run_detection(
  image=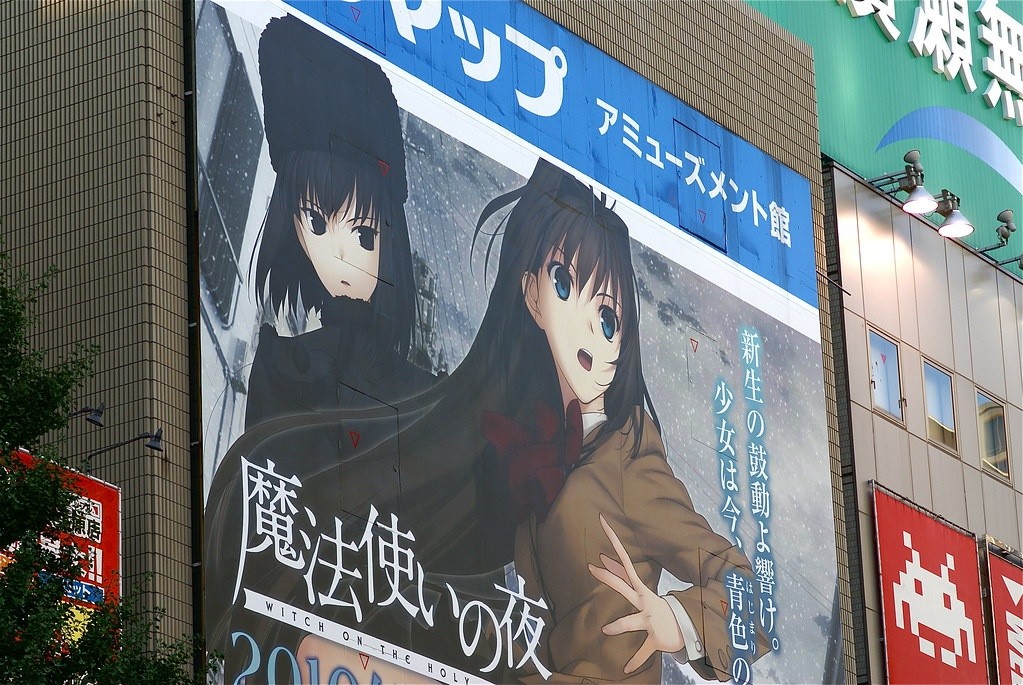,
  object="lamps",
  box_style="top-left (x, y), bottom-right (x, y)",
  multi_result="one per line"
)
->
top-left (999, 253), bottom-right (1023, 270)
top-left (867, 149), bottom-right (938, 214)
top-left (85, 426), bottom-right (164, 475)
top-left (64, 402), bottom-right (105, 427)
top-left (978, 209), bottom-right (1016, 253)
top-left (932, 189), bottom-right (974, 238)
